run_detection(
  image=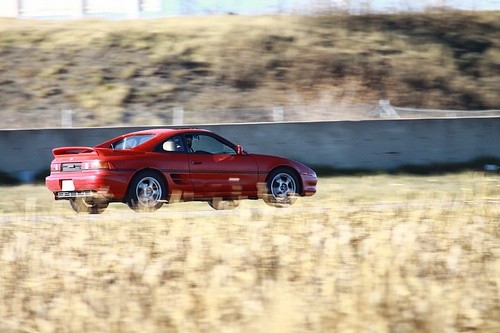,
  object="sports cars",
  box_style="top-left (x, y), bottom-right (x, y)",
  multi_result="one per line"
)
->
top-left (45, 128), bottom-right (318, 215)
